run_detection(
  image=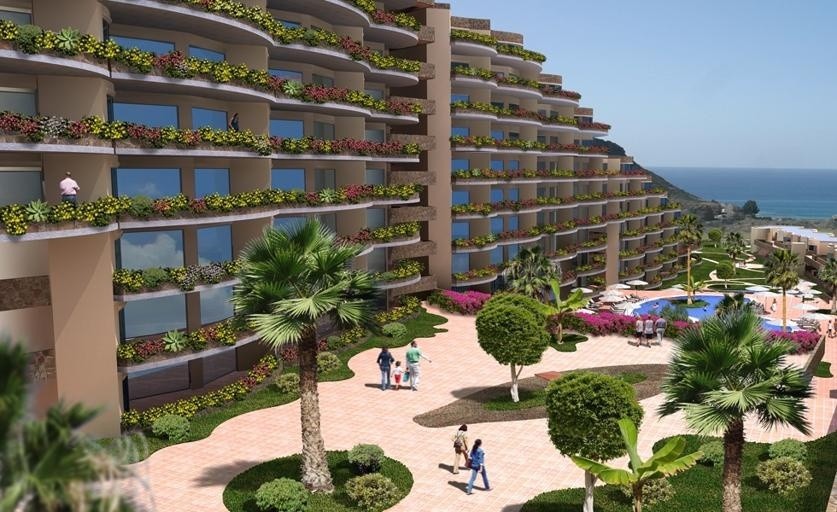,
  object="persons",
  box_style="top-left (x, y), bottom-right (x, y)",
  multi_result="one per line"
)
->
top-left (826, 320), bottom-right (833, 337)
top-left (377, 347), bottom-right (395, 390)
top-left (635, 315), bottom-right (644, 347)
top-left (405, 341), bottom-right (432, 391)
top-left (644, 314), bottom-right (655, 348)
top-left (450, 424), bottom-right (471, 474)
top-left (833, 318), bottom-right (837, 336)
top-left (465, 439), bottom-right (494, 494)
top-left (391, 361), bottom-right (405, 390)
top-left (655, 315), bottom-right (667, 345)
top-left (228, 112), bottom-right (239, 133)
top-left (59, 171), bottom-right (80, 204)
